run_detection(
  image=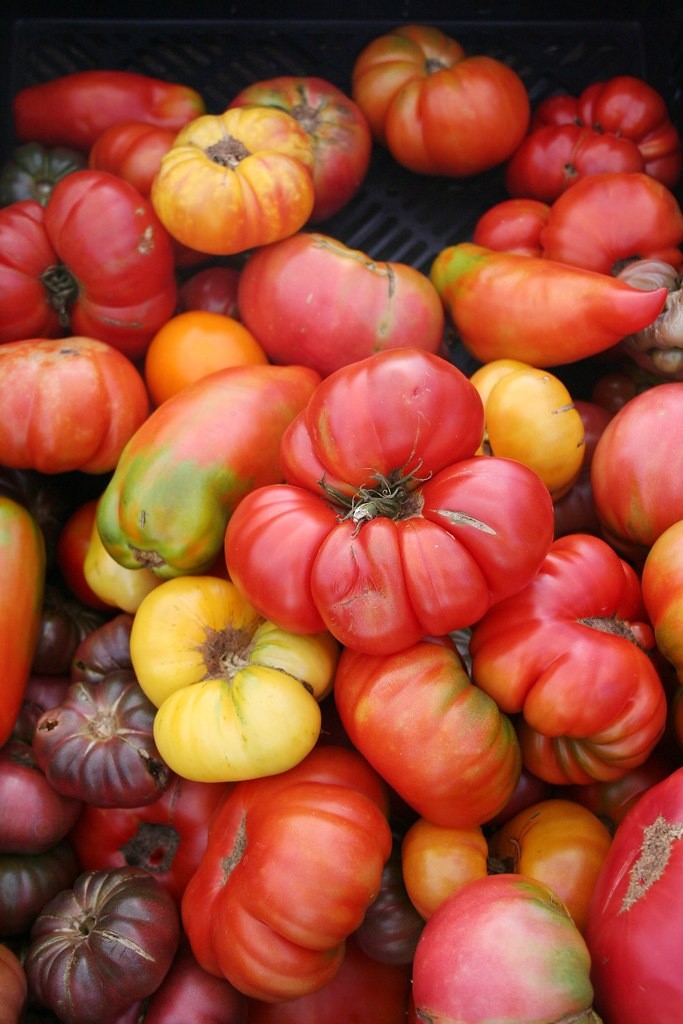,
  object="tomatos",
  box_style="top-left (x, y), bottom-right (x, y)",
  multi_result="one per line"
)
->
top-left (0, 24), bottom-right (683, 1024)
